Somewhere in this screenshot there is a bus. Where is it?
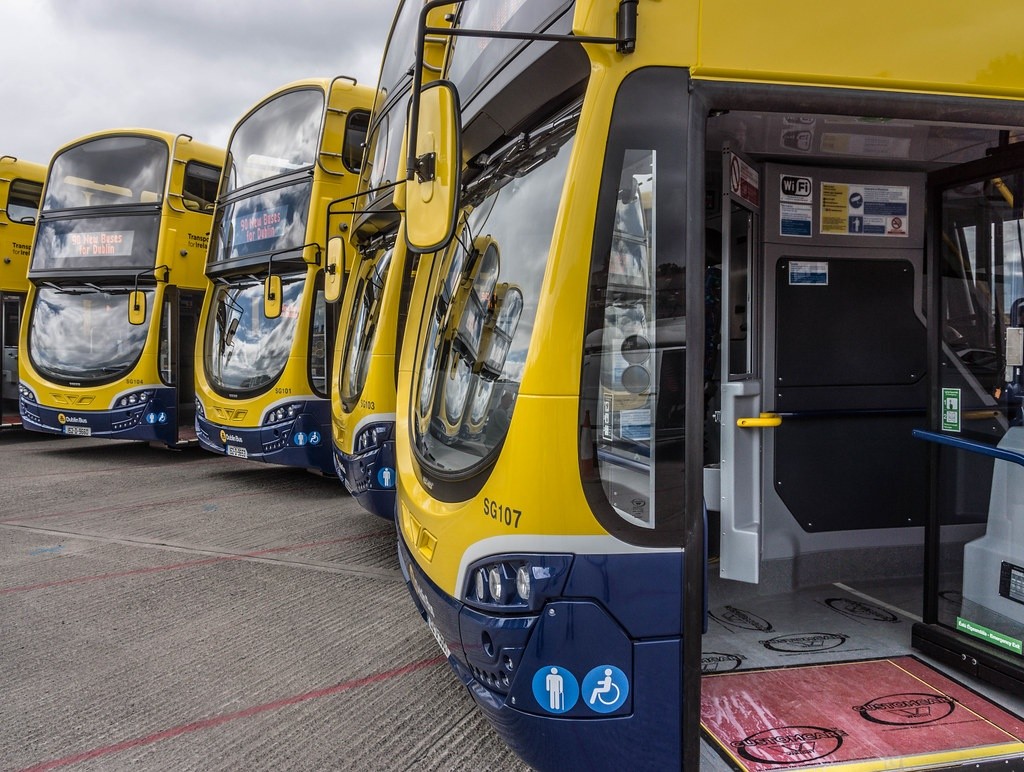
[191,75,378,492]
[391,1,1024,772]
[0,154,162,430]
[412,204,525,448]
[11,127,228,445]
[314,0,464,525]
